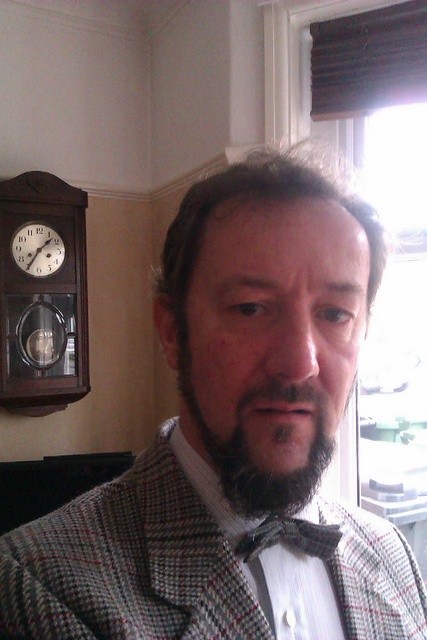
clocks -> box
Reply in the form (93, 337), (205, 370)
(0, 169), (91, 411)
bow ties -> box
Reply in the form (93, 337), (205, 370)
(234, 517), (343, 563)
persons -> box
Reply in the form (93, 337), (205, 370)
(0, 136), (426, 640)
